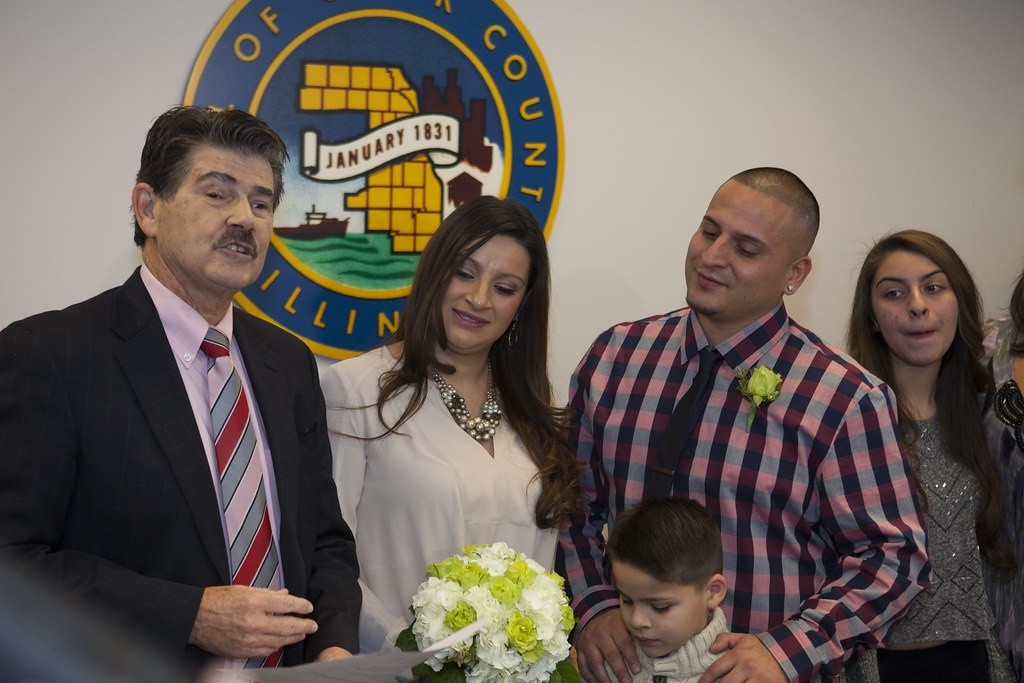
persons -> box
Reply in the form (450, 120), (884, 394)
(579, 494), (733, 683)
(0, 105), (364, 683)
(320, 194), (583, 683)
(846, 229), (1024, 683)
(554, 166), (935, 683)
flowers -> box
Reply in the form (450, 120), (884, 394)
(733, 364), (783, 435)
(393, 544), (583, 683)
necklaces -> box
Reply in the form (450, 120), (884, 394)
(429, 356), (503, 442)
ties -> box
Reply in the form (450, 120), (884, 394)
(641, 347), (724, 502)
(199, 327), (284, 669)
